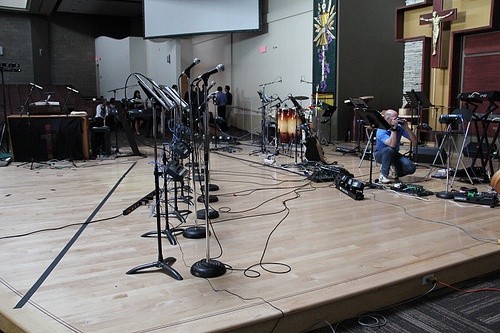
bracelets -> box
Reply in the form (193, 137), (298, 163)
(390, 128), (397, 131)
(405, 128), (410, 131)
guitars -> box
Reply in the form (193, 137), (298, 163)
(289, 97), (324, 161)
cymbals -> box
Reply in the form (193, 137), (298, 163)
(294, 96), (309, 100)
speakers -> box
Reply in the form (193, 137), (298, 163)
(463, 142), (497, 159)
(413, 147), (447, 164)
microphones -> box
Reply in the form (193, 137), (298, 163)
(30, 83), (43, 89)
(195, 64), (224, 81)
(67, 86), (79, 93)
(207, 81), (216, 91)
(198, 93), (215, 110)
(181, 58), (201, 76)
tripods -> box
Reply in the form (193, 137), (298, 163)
(344, 96), (374, 156)
(126, 75), (191, 280)
(354, 107), (395, 189)
(249, 80), (295, 159)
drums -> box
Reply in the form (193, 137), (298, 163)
(277, 107), (302, 144)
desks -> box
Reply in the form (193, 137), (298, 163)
(6, 114), (93, 162)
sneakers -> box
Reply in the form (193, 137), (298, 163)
(379, 173), (391, 184)
(389, 166), (398, 178)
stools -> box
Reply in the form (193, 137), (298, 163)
(425, 108), (474, 188)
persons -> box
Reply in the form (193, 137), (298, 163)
(172, 85), (177, 92)
(130, 90), (144, 135)
(216, 85), (232, 131)
(184, 84), (204, 117)
(95, 98), (120, 127)
(375, 109), (417, 184)
